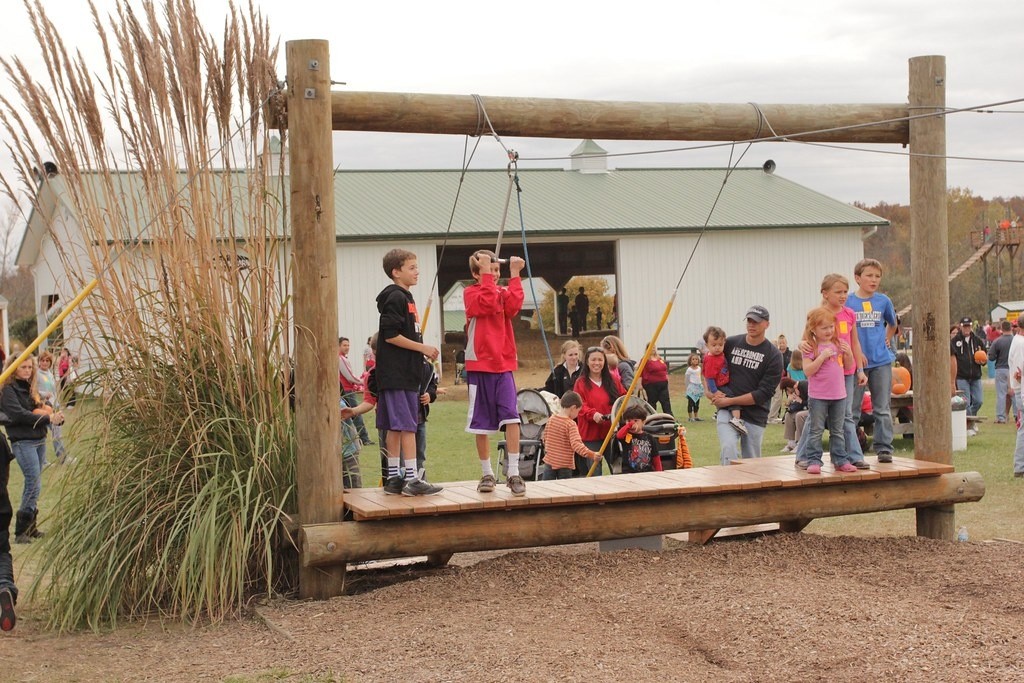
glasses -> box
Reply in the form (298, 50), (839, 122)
(586, 346), (604, 354)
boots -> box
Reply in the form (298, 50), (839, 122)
(27, 507), (45, 537)
(14, 510), (34, 544)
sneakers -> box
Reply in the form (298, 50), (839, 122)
(0, 587), (16, 631)
(383, 476), (405, 494)
(506, 474), (526, 496)
(477, 475), (496, 492)
(401, 468), (444, 497)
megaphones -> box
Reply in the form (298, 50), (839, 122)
(763, 160), (776, 174)
(33, 166), (43, 181)
(44, 162), (57, 178)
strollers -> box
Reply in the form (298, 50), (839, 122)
(494, 387), (560, 482)
(601, 394), (691, 473)
(453, 348), (468, 385)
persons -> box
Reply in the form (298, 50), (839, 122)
(0, 389), (18, 631)
(714, 304), (784, 465)
(0, 344), (6, 375)
(607, 294), (617, 329)
(846, 258), (898, 462)
(521, 339), (586, 478)
(35, 348), (79, 465)
(638, 342), (674, 416)
(0, 350), (64, 544)
(951, 314), (1024, 477)
(606, 352), (621, 381)
(802, 308), (858, 473)
(600, 335), (636, 473)
(281, 335), (437, 490)
(541, 389), (603, 481)
(700, 325), (748, 435)
(373, 248), (443, 497)
(463, 249), (526, 496)
(766, 309), (913, 452)
(684, 353), (705, 422)
(794, 273), (871, 469)
(616, 404), (662, 473)
(573, 346), (627, 476)
(557, 286), (590, 337)
(595, 307), (602, 330)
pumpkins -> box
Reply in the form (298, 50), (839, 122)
(974, 346), (986, 363)
(891, 361), (912, 395)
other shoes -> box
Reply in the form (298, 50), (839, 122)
(67, 405), (74, 409)
(687, 412), (800, 454)
(807, 464), (821, 474)
(43, 462), (54, 469)
(851, 459), (870, 469)
(65, 457), (77, 464)
(834, 462), (857, 472)
(967, 413), (1024, 477)
(729, 417), (748, 434)
(878, 451), (892, 462)
(794, 460), (808, 470)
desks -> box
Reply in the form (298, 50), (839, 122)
(863, 389), (963, 420)
(655, 347), (700, 375)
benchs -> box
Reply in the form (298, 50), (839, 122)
(655, 354), (701, 375)
(865, 411), (987, 436)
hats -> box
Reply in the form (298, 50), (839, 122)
(960, 316), (972, 326)
(743, 305), (769, 323)
